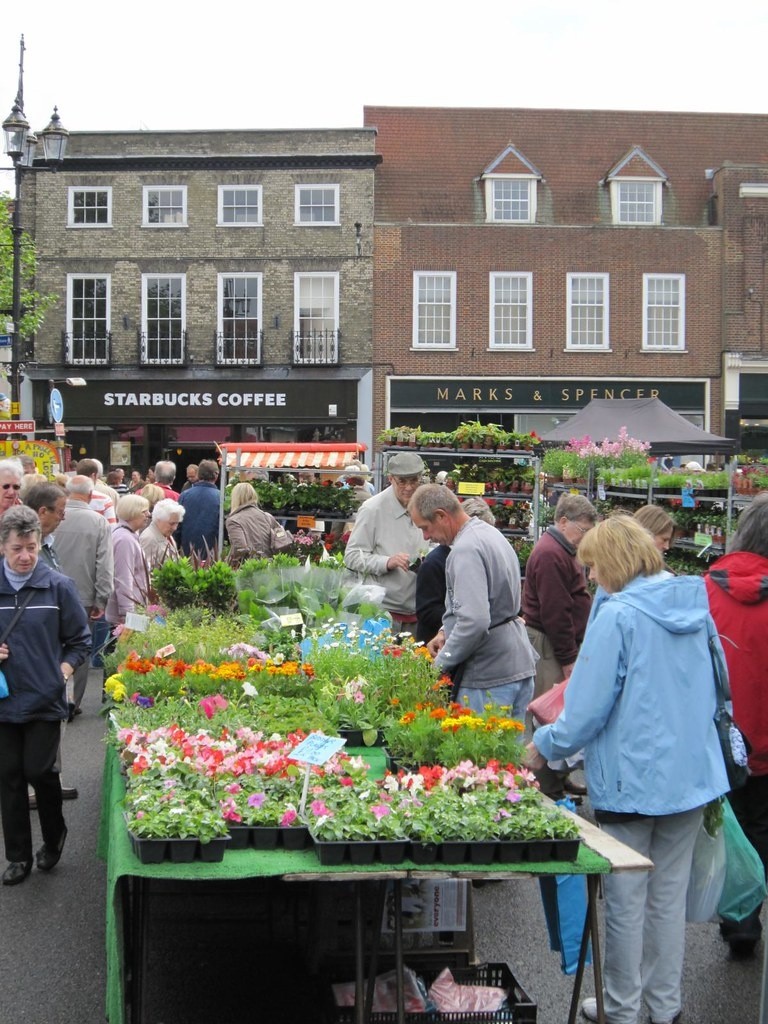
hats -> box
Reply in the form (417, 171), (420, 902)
(387, 452), (425, 476)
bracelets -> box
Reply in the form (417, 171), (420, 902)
(62, 673), (68, 680)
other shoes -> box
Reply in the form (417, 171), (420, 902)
(2, 857), (34, 886)
(582, 997), (599, 1021)
(68, 701), (78, 724)
(62, 787), (78, 801)
(28, 794), (37, 808)
(36, 825), (68, 870)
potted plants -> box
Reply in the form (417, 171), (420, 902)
(376, 420), (541, 451)
(663, 547), (725, 576)
(506, 537), (534, 566)
(446, 463), (536, 493)
(542, 448), (745, 545)
(223, 475), (366, 519)
(149, 534), (382, 616)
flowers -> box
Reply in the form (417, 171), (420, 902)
(564, 426), (652, 457)
(732, 454), (768, 495)
(290, 529), (352, 566)
(98, 604), (583, 867)
(530, 431), (543, 441)
(457, 496), (533, 518)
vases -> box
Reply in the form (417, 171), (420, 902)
(495, 518), (530, 530)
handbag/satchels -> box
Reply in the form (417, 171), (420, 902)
(685, 823), (727, 924)
(717, 795), (767, 922)
(713, 711), (753, 792)
(529, 678), (569, 725)
(270, 527), (295, 551)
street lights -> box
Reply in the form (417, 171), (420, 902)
(0, 92), (70, 441)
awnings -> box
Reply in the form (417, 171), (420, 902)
(213, 442), (368, 468)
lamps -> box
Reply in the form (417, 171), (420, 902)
(79, 444), (86, 454)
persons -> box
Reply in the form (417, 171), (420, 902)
(0, 505), (93, 886)
(523, 513), (750, 1024)
(345, 452), (431, 645)
(521, 493), (597, 805)
(588, 505), (678, 623)
(702, 490), (768, 943)
(225, 483), (284, 571)
(435, 470), (449, 485)
(415, 497), (495, 645)
(0, 455), (222, 810)
(299, 469), (315, 484)
(408, 483), (541, 745)
(335, 464), (374, 511)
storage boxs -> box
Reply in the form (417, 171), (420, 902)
(327, 964), (538, 1024)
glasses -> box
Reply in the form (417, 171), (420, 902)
(392, 476), (420, 488)
(46, 507), (66, 518)
(1, 483), (21, 490)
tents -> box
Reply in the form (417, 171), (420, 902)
(534, 397), (743, 556)
